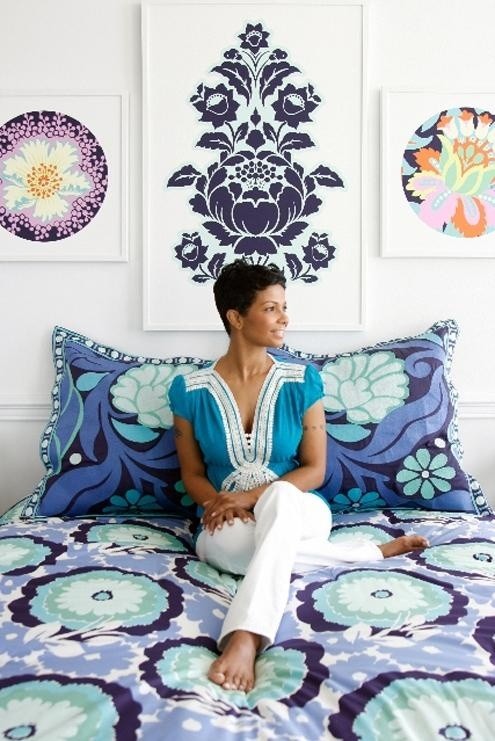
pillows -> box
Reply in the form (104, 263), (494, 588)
(17, 323), (212, 523)
(278, 319), (492, 517)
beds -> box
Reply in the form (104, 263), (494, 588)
(0, 480), (495, 741)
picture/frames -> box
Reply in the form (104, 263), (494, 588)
(0, 92), (130, 266)
(378, 84), (495, 261)
(140, 0), (369, 335)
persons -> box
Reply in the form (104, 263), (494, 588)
(167, 263), (431, 694)
